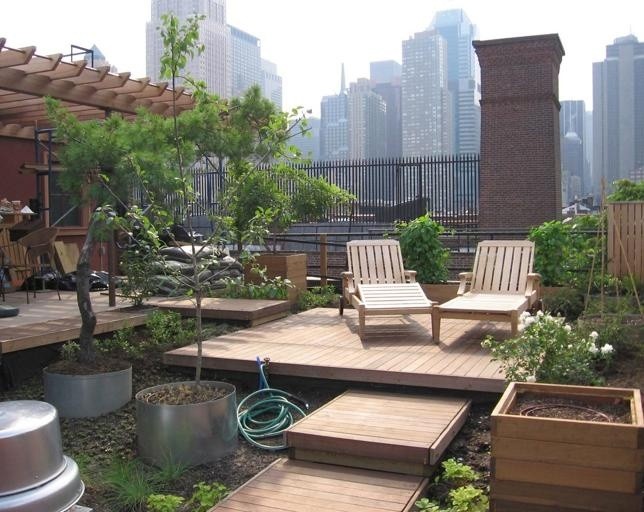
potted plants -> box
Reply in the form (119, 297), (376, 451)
(213, 164), (359, 304)
(488, 383), (644, 496)
(584, 272), (644, 313)
(381, 215), (578, 311)
(88, 12), (295, 470)
(577, 291), (644, 333)
(45, 88), (318, 420)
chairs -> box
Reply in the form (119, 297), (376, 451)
(339, 239), (439, 342)
(433, 239), (545, 346)
(0, 226), (61, 304)
